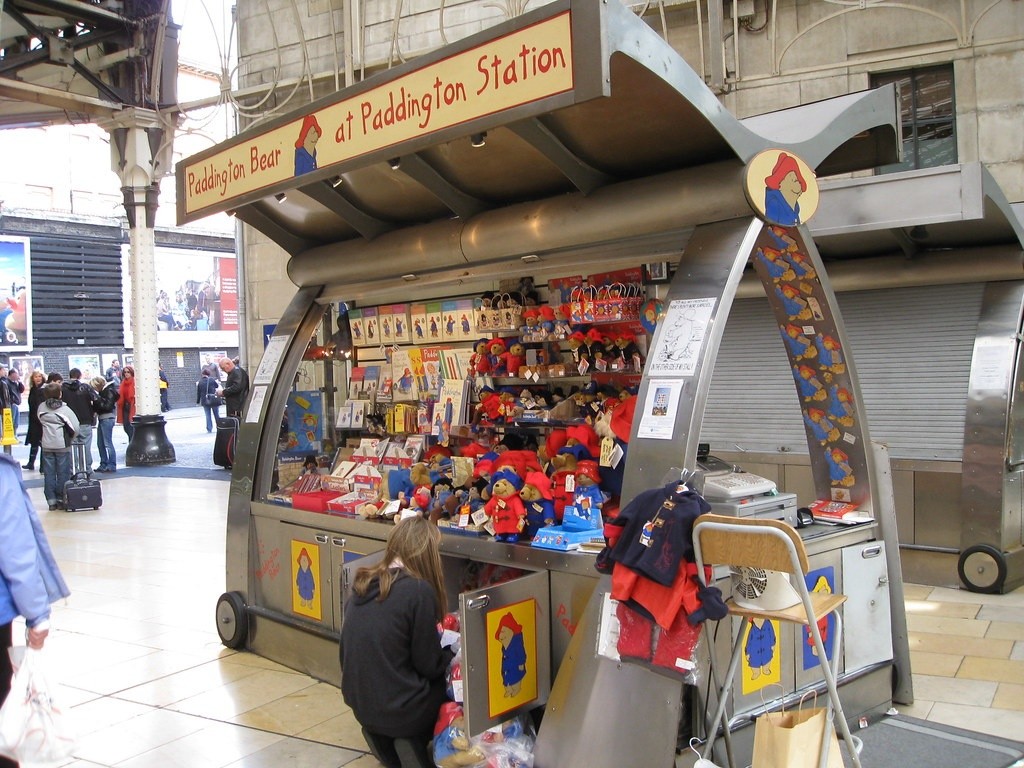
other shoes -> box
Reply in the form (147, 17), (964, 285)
(103, 466), (117, 472)
(23, 464), (35, 470)
(394, 737), (425, 768)
(48, 505), (57, 511)
(94, 466), (106, 472)
(58, 502), (67, 510)
(361, 725), (393, 766)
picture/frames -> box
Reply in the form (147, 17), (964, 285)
(641, 261), (671, 286)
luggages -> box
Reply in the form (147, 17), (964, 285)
(213, 408), (242, 469)
(63, 442), (102, 513)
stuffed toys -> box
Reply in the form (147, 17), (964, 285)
(357, 277), (645, 543)
(430, 558), (533, 768)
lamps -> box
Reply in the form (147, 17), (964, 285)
(327, 175), (343, 188)
(275, 193), (287, 204)
(387, 157), (401, 170)
(471, 130), (488, 147)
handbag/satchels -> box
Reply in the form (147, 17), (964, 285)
(751, 683), (846, 768)
(0, 617), (75, 768)
(205, 394), (222, 407)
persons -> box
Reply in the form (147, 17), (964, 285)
(156, 278), (220, 331)
(159, 362), (171, 412)
(216, 357), (249, 417)
(0, 451), (71, 768)
(202, 354), (220, 379)
(196, 369), (220, 432)
(12, 360), (41, 386)
(106, 359), (124, 392)
(0, 364), (135, 479)
(37, 383), (80, 510)
(338, 516), (461, 768)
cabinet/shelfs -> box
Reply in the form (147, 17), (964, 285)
(475, 316), (643, 430)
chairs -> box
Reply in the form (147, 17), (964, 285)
(692, 514), (863, 768)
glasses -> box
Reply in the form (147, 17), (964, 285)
(123, 371), (130, 374)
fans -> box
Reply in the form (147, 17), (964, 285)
(728, 562), (803, 611)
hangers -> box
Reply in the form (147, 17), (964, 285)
(689, 737), (721, 768)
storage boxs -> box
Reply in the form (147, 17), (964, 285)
(548, 364), (573, 377)
(519, 366), (530, 379)
(530, 366), (548, 378)
